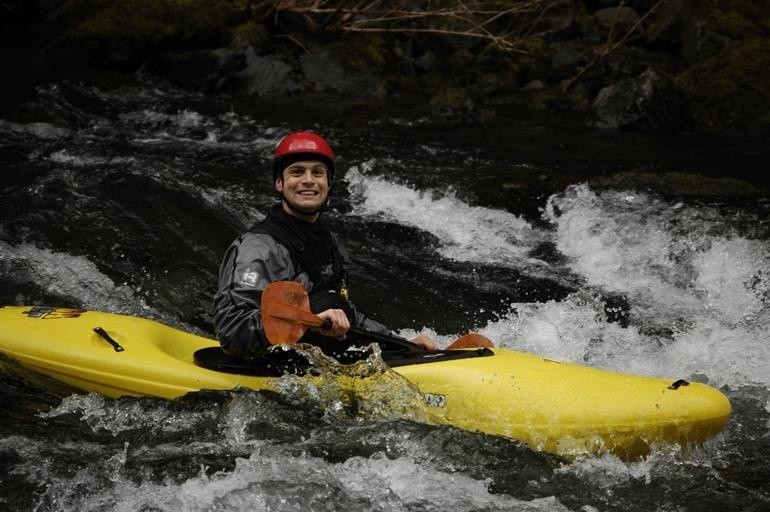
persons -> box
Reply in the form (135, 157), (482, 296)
(213, 132), (436, 356)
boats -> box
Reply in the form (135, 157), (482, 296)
(0, 305), (733, 463)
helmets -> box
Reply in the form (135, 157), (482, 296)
(272, 130), (336, 181)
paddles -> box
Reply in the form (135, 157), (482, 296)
(260, 280), (494, 351)
